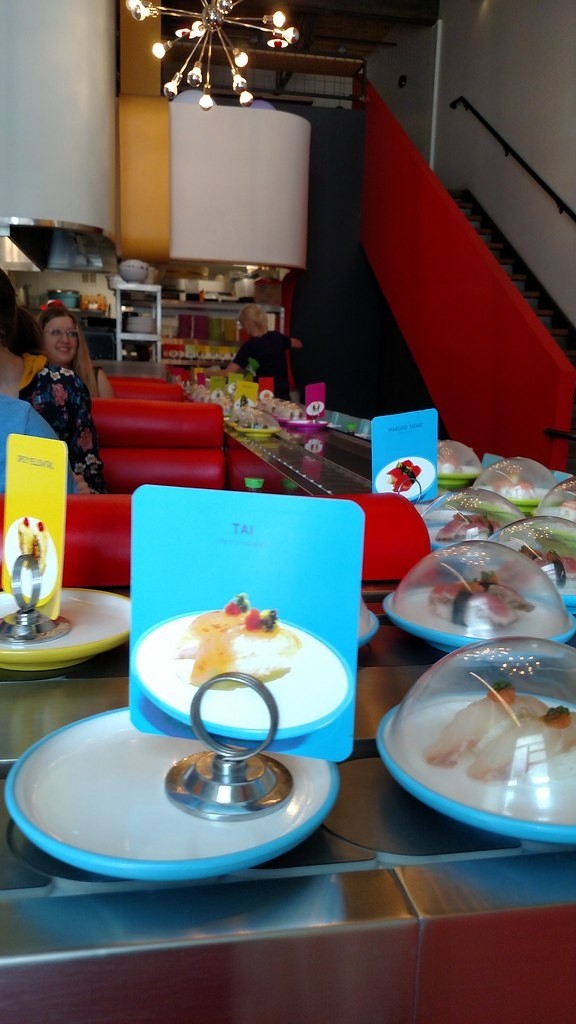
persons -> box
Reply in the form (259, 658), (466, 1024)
(201, 304), (303, 402)
(0, 268), (111, 495)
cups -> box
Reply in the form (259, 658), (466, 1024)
(224, 315), (238, 342)
(210, 317), (223, 342)
(195, 314), (209, 341)
(177, 313), (193, 339)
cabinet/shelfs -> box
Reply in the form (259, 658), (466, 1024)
(160, 300), (285, 373)
(115, 282), (161, 364)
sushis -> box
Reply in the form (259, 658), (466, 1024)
(175, 595), (250, 660)
(495, 483), (513, 497)
(429, 582), (518, 628)
(29, 522), (48, 575)
(394, 464), (421, 492)
(466, 571), (535, 612)
(18, 518), (30, 566)
(560, 500), (576, 520)
(518, 545), (543, 560)
(386, 460), (413, 484)
(183, 381), (303, 429)
(537, 550), (576, 588)
(468, 705), (576, 782)
(457, 513), (501, 539)
(512, 482), (533, 498)
(424, 681), (549, 767)
(436, 515), (472, 543)
(191, 608), (299, 690)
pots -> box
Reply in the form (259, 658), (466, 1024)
(118, 258), (152, 286)
(47, 288), (79, 309)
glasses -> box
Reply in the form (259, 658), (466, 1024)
(43, 328), (78, 337)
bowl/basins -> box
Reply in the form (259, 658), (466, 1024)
(127, 318), (156, 334)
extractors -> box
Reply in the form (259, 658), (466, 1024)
(8, 221), (120, 274)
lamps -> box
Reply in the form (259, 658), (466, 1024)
(125, 0), (300, 112)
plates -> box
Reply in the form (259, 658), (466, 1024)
(506, 498), (541, 517)
(130, 610), (354, 741)
(437, 466), (483, 490)
(224, 417), (282, 438)
(3, 707), (338, 882)
(358, 596), (576, 653)
(374, 702), (576, 843)
(418, 505), (576, 613)
(273, 415), (329, 431)
(0, 587), (130, 671)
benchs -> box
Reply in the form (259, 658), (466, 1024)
(0, 492), (431, 599)
(110, 382), (183, 401)
(107, 377), (167, 387)
(91, 399), (226, 493)
